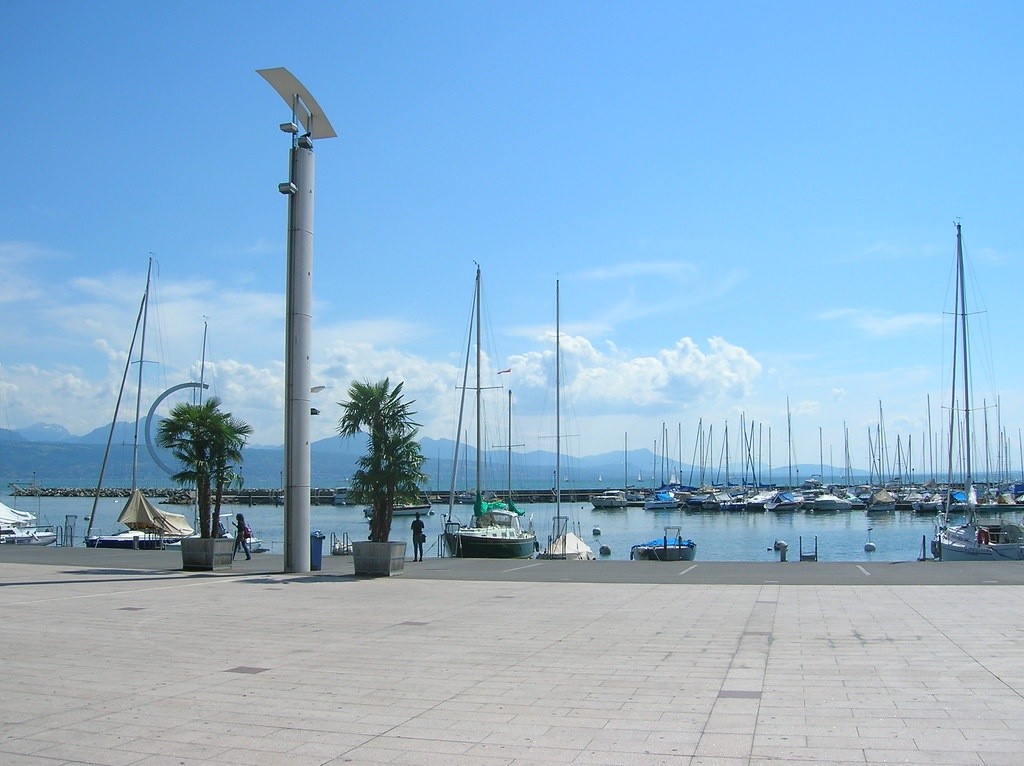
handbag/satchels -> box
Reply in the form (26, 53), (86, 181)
(422, 534), (426, 543)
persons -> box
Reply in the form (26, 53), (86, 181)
(410, 512), (425, 562)
(367, 509), (374, 540)
(232, 513), (251, 560)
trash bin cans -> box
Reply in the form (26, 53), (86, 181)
(310, 529), (326, 571)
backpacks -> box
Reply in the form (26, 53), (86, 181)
(244, 524), (252, 539)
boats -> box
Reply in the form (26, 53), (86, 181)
(632, 526), (696, 561)
(362, 496), (431, 517)
(0, 521), (57, 545)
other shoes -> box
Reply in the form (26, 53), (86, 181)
(419, 560), (422, 561)
(413, 560), (417, 562)
(246, 556), (251, 560)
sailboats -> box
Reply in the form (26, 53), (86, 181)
(83, 251), (193, 548)
(164, 315), (261, 553)
(766, 395), (942, 513)
(537, 279), (595, 560)
(933, 221), (1024, 559)
(942, 396), (1024, 511)
(454, 431), (479, 503)
(442, 261), (538, 558)
(589, 411), (777, 511)
